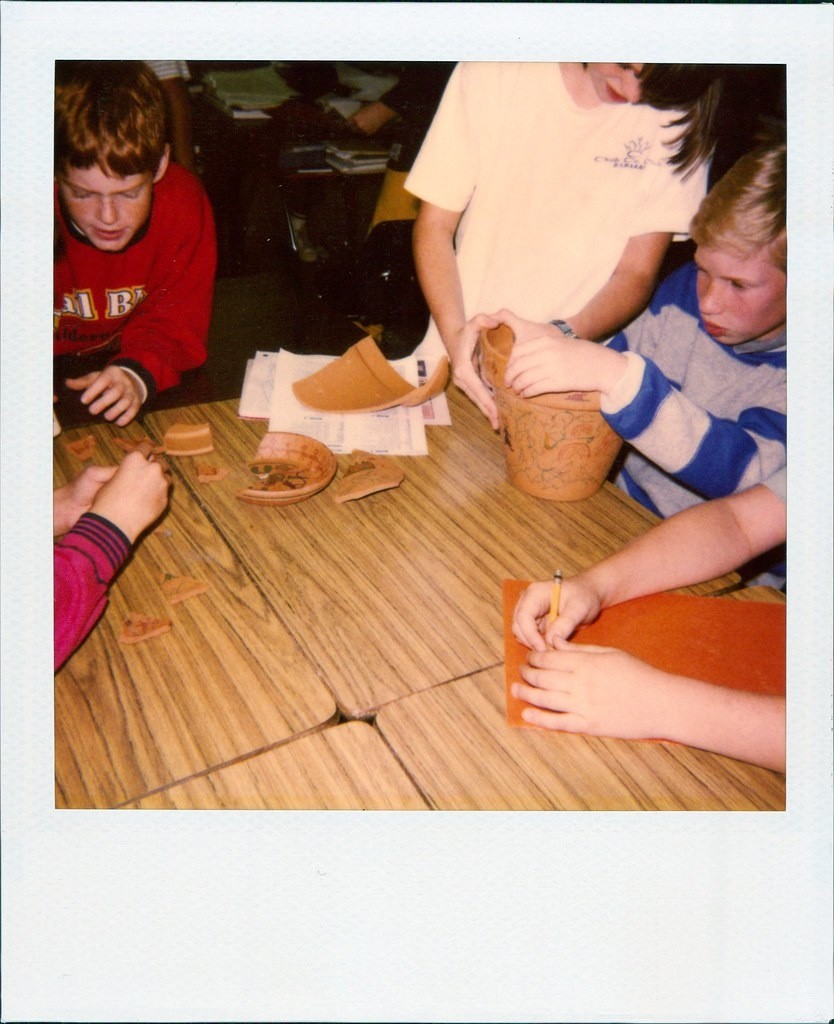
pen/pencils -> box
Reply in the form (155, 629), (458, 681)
(546, 569), (563, 651)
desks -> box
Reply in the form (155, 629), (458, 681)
(54, 384), (786, 811)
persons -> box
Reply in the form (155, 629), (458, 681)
(54, 60), (218, 437)
(287, 61), (457, 151)
(511, 466), (786, 776)
(53, 437), (173, 673)
(402, 62), (727, 430)
(505, 136), (786, 598)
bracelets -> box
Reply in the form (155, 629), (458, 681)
(548, 319), (581, 339)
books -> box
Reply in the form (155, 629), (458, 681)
(204, 94), (274, 119)
(324, 141), (389, 174)
